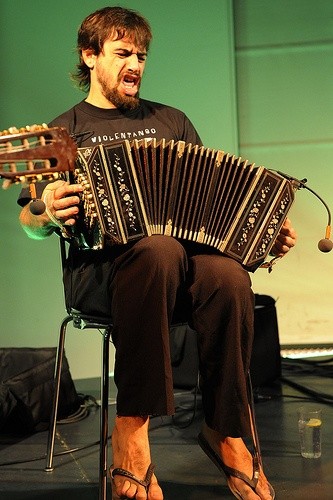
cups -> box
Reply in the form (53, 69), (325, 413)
(298, 408), (323, 458)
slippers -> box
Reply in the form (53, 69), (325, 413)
(196, 431), (276, 500)
(109, 463), (157, 500)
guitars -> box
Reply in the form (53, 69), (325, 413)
(0, 120), (95, 191)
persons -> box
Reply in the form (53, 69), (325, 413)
(17, 5), (296, 499)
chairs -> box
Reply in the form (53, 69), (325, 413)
(43, 234), (264, 500)
